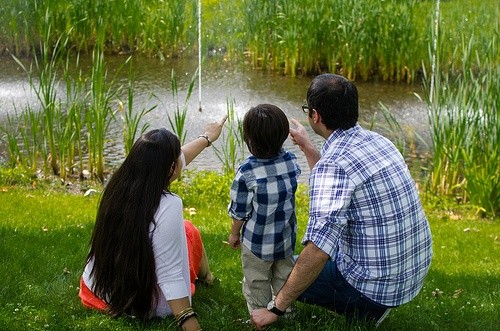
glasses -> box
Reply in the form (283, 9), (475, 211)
(302, 103), (313, 113)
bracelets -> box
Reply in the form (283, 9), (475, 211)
(199, 135), (212, 147)
(176, 308), (195, 326)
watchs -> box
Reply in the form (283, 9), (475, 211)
(267, 300), (286, 317)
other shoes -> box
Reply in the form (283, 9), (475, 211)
(198, 272), (214, 288)
(345, 296), (391, 329)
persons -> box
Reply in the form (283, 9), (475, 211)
(78, 114), (229, 331)
(229, 105), (302, 316)
(250, 74), (432, 331)
